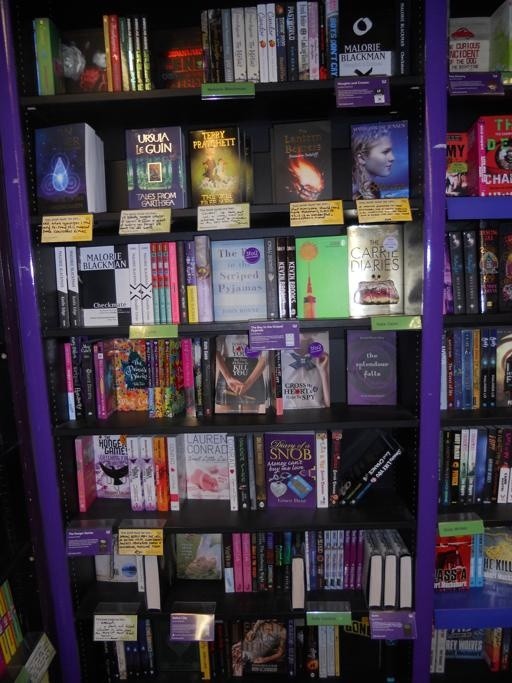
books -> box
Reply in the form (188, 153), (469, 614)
(0, 0), (511, 682)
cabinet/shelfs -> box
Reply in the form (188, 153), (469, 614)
(0, 0), (512, 683)
